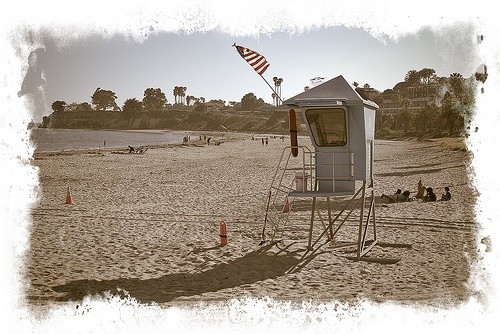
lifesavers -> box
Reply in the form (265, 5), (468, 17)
(289, 109), (298, 157)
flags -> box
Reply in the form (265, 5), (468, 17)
(236, 45), (270, 75)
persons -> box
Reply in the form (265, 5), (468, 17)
(423, 187), (436, 202)
(441, 187), (452, 200)
(183, 137), (188, 143)
(128, 145), (134, 154)
(266, 138), (269, 146)
(261, 138), (265, 146)
(282, 136), (284, 142)
(252, 135), (255, 141)
(380, 189), (412, 203)
(207, 137), (212, 146)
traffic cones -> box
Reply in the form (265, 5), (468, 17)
(64, 186), (74, 204)
